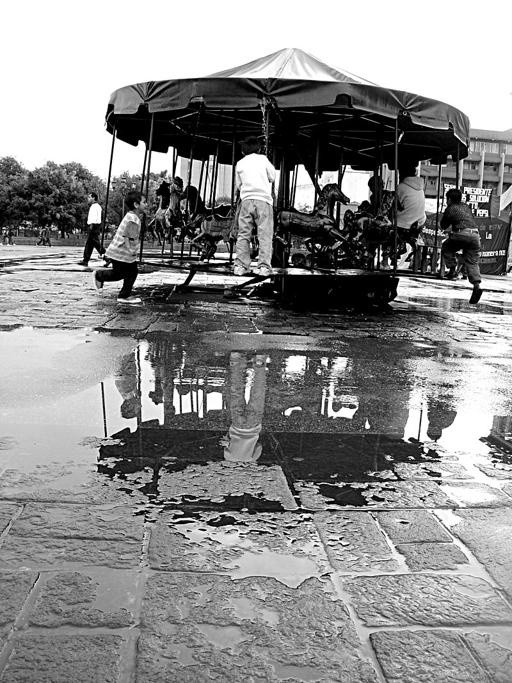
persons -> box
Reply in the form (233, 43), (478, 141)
(220, 352), (266, 462)
(233, 136), (277, 277)
(393, 161), (427, 255)
(92, 191), (148, 304)
(114, 353), (141, 419)
(76, 191), (112, 268)
(0, 218), (82, 246)
(427, 401), (457, 440)
(440, 189), (483, 304)
(355, 177), (393, 222)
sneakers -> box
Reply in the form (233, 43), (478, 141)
(469, 286), (482, 304)
(94, 271), (104, 290)
(117, 296), (141, 303)
(234, 266), (251, 275)
(259, 267), (272, 276)
(444, 270), (457, 277)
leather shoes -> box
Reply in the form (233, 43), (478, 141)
(77, 260), (88, 267)
(104, 260), (111, 267)
